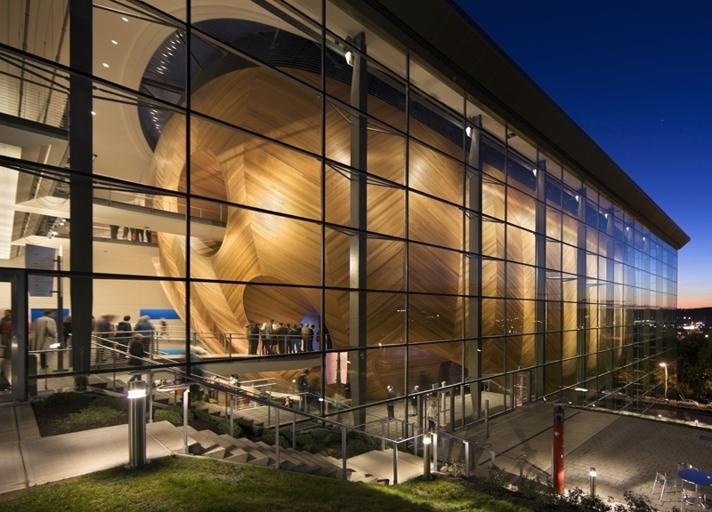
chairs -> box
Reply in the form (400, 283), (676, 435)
(652, 462), (712, 512)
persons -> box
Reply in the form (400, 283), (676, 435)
(127, 334), (151, 382)
(213, 374), (241, 388)
(344, 381), (351, 398)
(427, 401), (439, 433)
(109, 225), (153, 244)
(62, 314), (167, 363)
(31, 310), (58, 369)
(0, 310), (12, 383)
(297, 369), (310, 400)
(281, 396), (293, 408)
(247, 319), (327, 355)
(408, 385), (420, 415)
(386, 386), (397, 421)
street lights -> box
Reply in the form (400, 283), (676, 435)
(659, 363), (669, 399)
